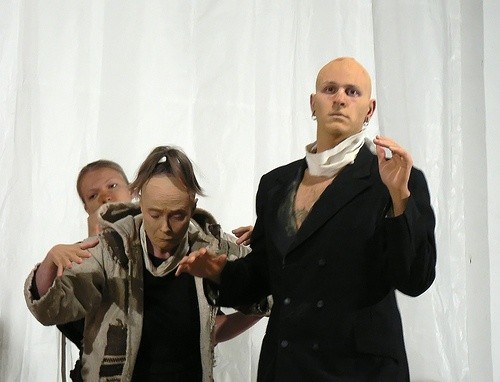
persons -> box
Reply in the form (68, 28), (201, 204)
(24, 58), (437, 382)
